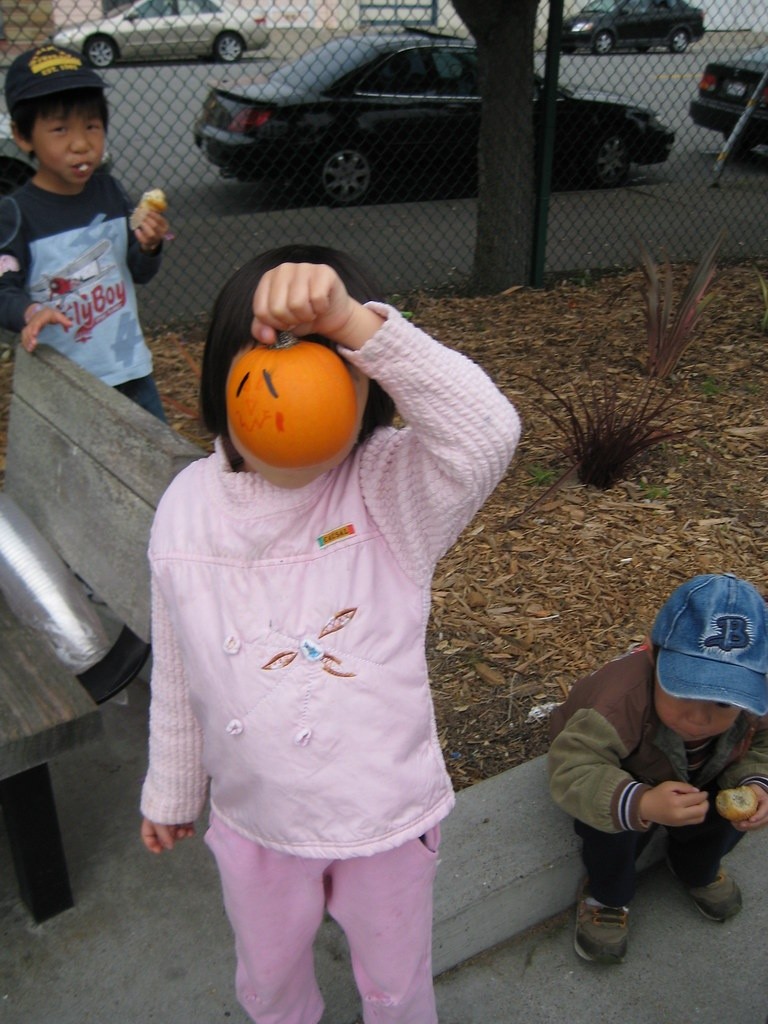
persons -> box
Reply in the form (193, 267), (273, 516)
(543, 571), (768, 966)
(135, 239), (523, 1024)
(0, 42), (163, 428)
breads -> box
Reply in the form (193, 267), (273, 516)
(716, 785), (758, 822)
(129, 189), (167, 231)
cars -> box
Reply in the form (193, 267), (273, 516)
(688, 43), (768, 152)
(191, 29), (678, 209)
(561, 0), (704, 55)
(1, 112), (114, 195)
(51, 0), (272, 70)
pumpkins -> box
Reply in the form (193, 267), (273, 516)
(225, 332), (356, 467)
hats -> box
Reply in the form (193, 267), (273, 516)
(652, 572), (768, 716)
(5, 43), (110, 111)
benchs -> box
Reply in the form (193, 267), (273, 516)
(0, 333), (207, 928)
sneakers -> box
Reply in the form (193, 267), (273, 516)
(574, 877), (629, 964)
(666, 857), (742, 923)
